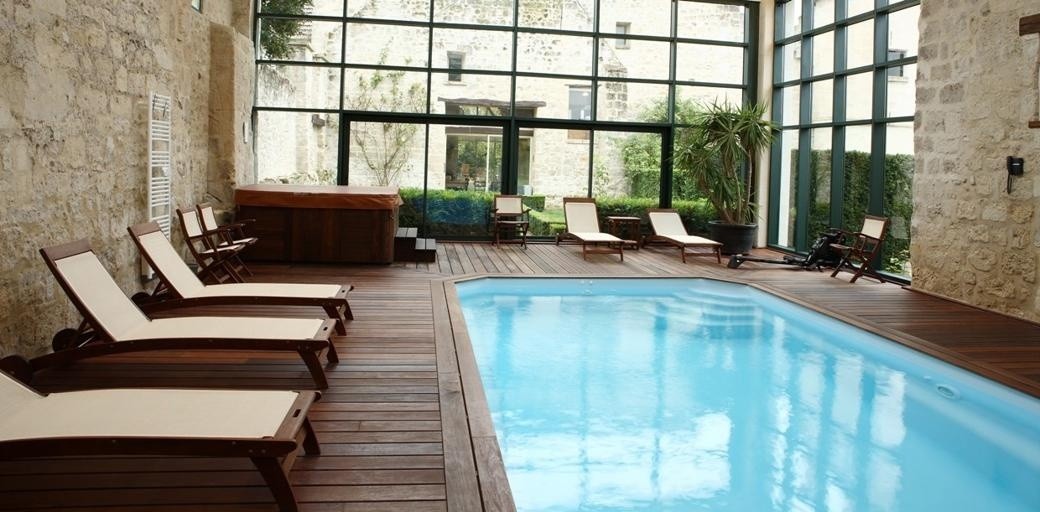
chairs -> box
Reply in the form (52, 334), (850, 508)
(1, 368), (327, 511)
(197, 202), (264, 280)
(128, 223), (356, 335)
(486, 193), (532, 250)
(642, 207), (723, 266)
(827, 214), (890, 284)
(556, 197), (626, 261)
(176, 208), (254, 284)
(39, 237), (340, 388)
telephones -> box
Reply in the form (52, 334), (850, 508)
(1006, 156), (1023, 176)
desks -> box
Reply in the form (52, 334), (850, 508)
(606, 215), (641, 250)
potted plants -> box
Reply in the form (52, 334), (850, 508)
(675, 88), (784, 257)
